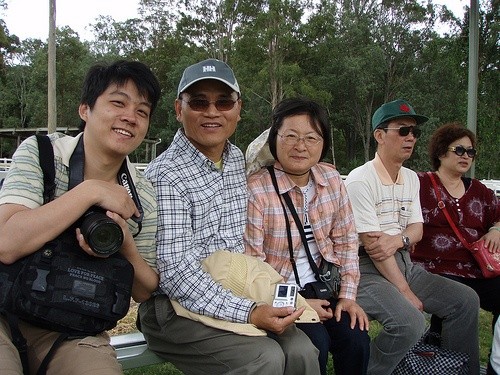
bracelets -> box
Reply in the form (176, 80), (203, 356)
(489, 227), (500, 231)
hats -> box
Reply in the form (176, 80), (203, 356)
(372, 99), (429, 131)
(176, 58), (241, 102)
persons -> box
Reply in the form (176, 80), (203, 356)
(143, 58), (320, 375)
(0, 60), (160, 375)
(343, 100), (480, 375)
(414, 123), (500, 375)
(244, 98), (370, 375)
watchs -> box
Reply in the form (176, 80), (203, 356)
(402, 235), (410, 245)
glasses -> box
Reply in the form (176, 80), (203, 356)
(276, 128), (324, 147)
(447, 146), (477, 158)
(179, 95), (241, 112)
(376, 126), (423, 139)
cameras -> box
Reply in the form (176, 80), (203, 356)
(273, 284), (298, 308)
(54, 205), (124, 256)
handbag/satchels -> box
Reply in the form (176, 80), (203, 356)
(469, 238), (500, 278)
(304, 260), (341, 311)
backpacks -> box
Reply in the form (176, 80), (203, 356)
(0, 134), (135, 375)
